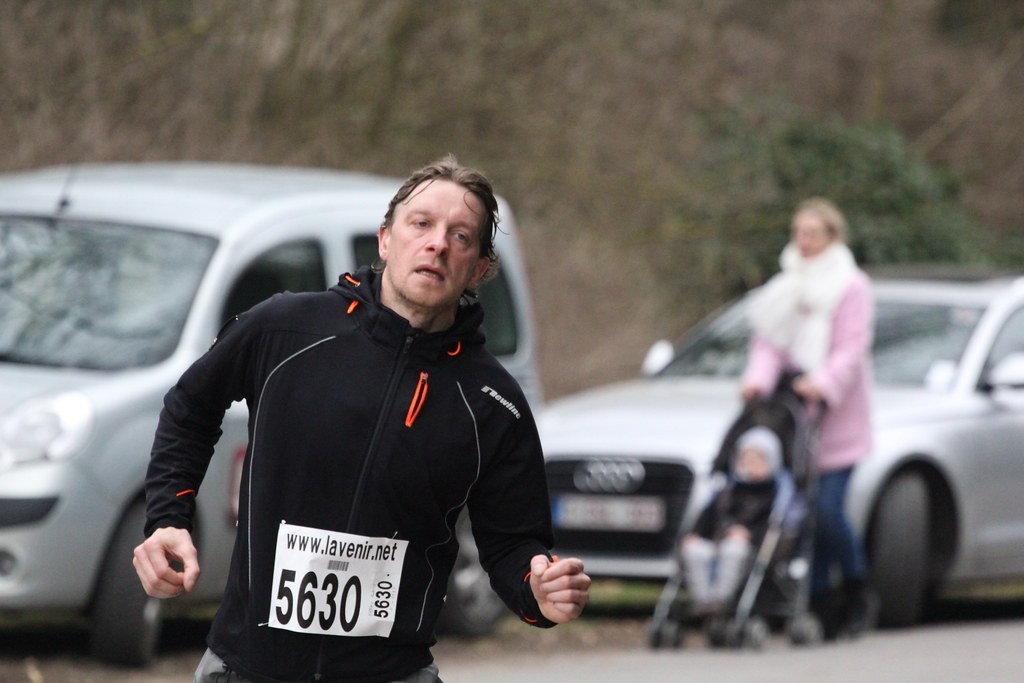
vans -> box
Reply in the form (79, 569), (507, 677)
(0, 147), (547, 674)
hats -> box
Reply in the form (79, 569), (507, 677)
(738, 425), (783, 473)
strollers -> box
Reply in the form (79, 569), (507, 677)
(643, 372), (827, 647)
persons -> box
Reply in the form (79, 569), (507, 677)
(681, 426), (785, 616)
(132, 155), (592, 683)
(741, 197), (874, 640)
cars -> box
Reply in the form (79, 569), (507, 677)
(534, 266), (1024, 629)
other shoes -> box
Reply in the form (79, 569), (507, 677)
(844, 575), (874, 633)
(810, 587), (838, 638)
(690, 595), (734, 616)
